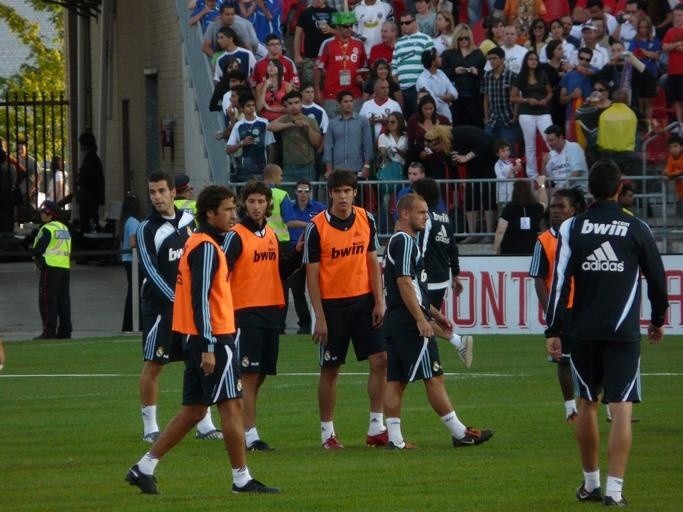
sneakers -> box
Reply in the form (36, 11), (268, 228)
(568, 412), (576, 423)
(453, 427), (492, 447)
(195, 429), (274, 450)
(143, 432), (159, 443)
(456, 336), (473, 368)
(232, 479), (280, 493)
(126, 465), (158, 493)
(576, 483), (627, 507)
(323, 430), (416, 449)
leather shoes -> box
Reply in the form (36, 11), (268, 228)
(34, 333), (70, 339)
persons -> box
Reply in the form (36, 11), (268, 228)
(121, 168), (491, 497)
(0, 336), (7, 369)
(185, 0), (682, 260)
(33, 201), (75, 338)
(0, 126), (108, 261)
(530, 161), (668, 505)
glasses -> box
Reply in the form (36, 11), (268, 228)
(297, 188), (309, 192)
(401, 20), (413, 25)
(579, 56), (589, 61)
(387, 120), (395, 123)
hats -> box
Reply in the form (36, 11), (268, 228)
(331, 13), (357, 26)
(581, 23), (596, 31)
(175, 175), (191, 190)
(40, 200), (60, 217)
